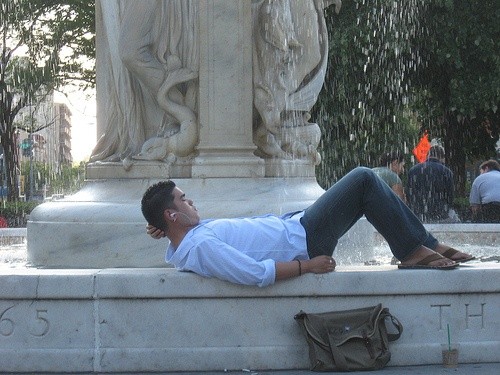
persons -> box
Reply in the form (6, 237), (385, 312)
(407, 145), (457, 222)
(141, 167), (476, 287)
(120, 0), (185, 137)
(373, 155), (406, 201)
(470, 160), (500, 223)
(251, 0), (329, 133)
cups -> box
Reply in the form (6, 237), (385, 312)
(441, 343), (461, 369)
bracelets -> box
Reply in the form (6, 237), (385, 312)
(296, 258), (301, 275)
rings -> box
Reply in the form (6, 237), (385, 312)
(329, 259), (332, 263)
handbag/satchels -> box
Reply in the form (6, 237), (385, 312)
(294, 302), (403, 372)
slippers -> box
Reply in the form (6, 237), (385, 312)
(398, 247), (475, 270)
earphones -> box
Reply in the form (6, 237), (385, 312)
(169, 213), (175, 218)
